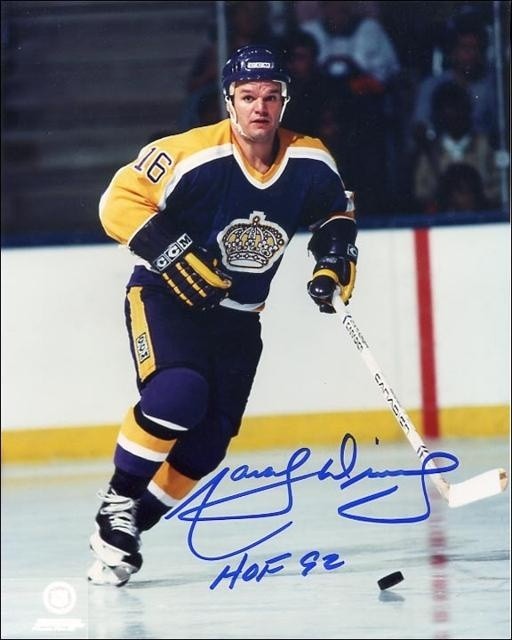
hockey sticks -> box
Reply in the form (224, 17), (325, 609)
(332, 285), (509, 510)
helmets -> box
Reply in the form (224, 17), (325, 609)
(221, 44), (292, 106)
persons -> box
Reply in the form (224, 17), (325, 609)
(94, 41), (359, 569)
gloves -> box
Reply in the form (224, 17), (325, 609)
(130, 213), (233, 313)
(307, 219), (359, 314)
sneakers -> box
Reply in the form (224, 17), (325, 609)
(93, 486), (142, 556)
(123, 533), (142, 568)
